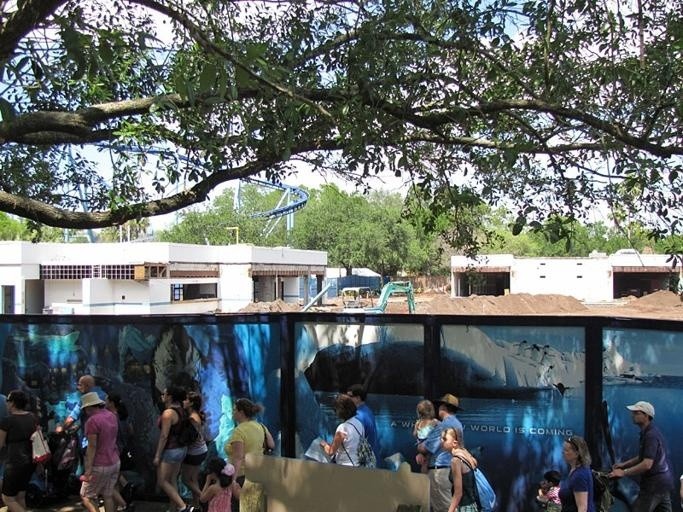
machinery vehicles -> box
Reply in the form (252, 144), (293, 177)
(340, 280), (415, 314)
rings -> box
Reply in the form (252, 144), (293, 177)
(610, 472), (613, 474)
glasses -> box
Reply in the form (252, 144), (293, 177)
(566, 436), (578, 450)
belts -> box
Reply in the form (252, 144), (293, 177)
(429, 466), (447, 469)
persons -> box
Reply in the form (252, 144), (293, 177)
(153, 385), (275, 511)
(556, 434), (595, 511)
(679, 474), (683, 510)
(534, 470), (563, 512)
(607, 399), (675, 511)
(413, 394), (478, 512)
(1, 375), (134, 512)
(320, 383), (376, 467)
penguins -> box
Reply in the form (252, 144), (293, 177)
(519, 340), (555, 378)
(552, 383), (570, 397)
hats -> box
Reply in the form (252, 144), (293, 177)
(347, 383), (368, 398)
(432, 393), (464, 411)
(626, 401), (654, 419)
(79, 391), (105, 410)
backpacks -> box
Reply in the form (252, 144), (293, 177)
(341, 421), (376, 468)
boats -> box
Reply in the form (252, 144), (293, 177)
(603, 373), (642, 386)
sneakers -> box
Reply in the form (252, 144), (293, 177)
(124, 481), (136, 500)
(176, 504), (195, 512)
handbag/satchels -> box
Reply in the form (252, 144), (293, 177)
(463, 450), (497, 512)
(31, 414), (53, 464)
(261, 423), (274, 456)
(173, 407), (199, 446)
(591, 469), (610, 512)
(120, 446), (138, 471)
(57, 430), (80, 473)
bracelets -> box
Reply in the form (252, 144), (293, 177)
(623, 468), (628, 475)
